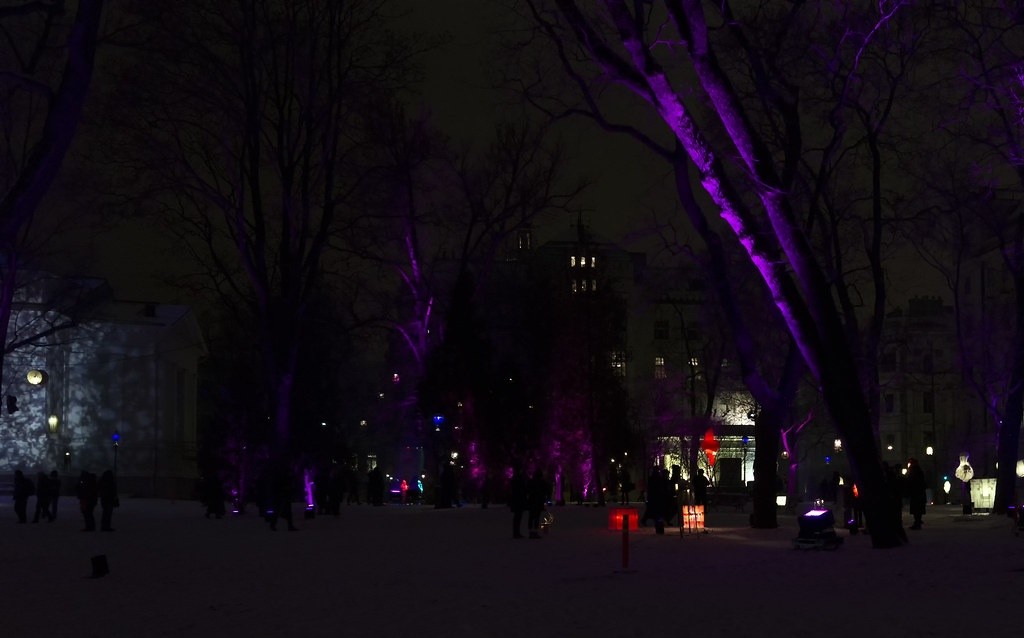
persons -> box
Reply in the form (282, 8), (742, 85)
(264, 466), (300, 531)
(13, 468), (61, 524)
(692, 468), (710, 514)
(642, 466), (675, 535)
(441, 462), (463, 508)
(843, 480), (864, 530)
(508, 463), (549, 540)
(344, 466), (387, 507)
(618, 467), (631, 505)
(199, 475), (226, 519)
(902, 458), (928, 530)
(78, 469), (120, 533)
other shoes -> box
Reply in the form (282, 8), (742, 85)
(288, 528), (299, 531)
(270, 525), (277, 531)
(528, 531), (542, 539)
(909, 525), (921, 530)
(102, 528), (115, 531)
(205, 513), (210, 519)
(81, 527), (95, 531)
(215, 516), (222, 519)
(918, 520), (924, 523)
(16, 510), (57, 524)
(513, 534), (523, 539)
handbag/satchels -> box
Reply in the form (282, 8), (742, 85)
(113, 498), (119, 507)
